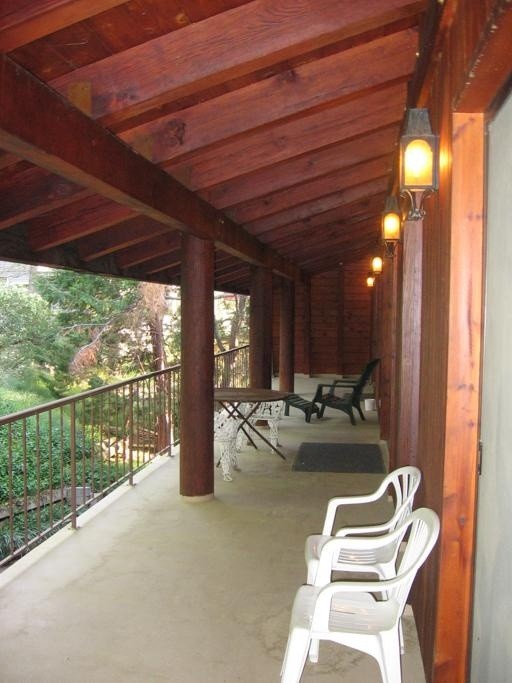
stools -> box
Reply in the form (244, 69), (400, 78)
(283, 392), (319, 421)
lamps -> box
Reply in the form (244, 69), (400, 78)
(378, 194), (401, 260)
(395, 104), (439, 221)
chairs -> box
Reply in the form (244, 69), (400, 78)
(279, 465), (441, 683)
(306, 356), (381, 425)
(212, 399), (286, 481)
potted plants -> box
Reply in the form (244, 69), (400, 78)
(361, 365), (377, 411)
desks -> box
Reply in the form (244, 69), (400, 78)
(214, 386), (290, 467)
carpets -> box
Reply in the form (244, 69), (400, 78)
(292, 441), (388, 474)
(342, 391), (374, 402)
